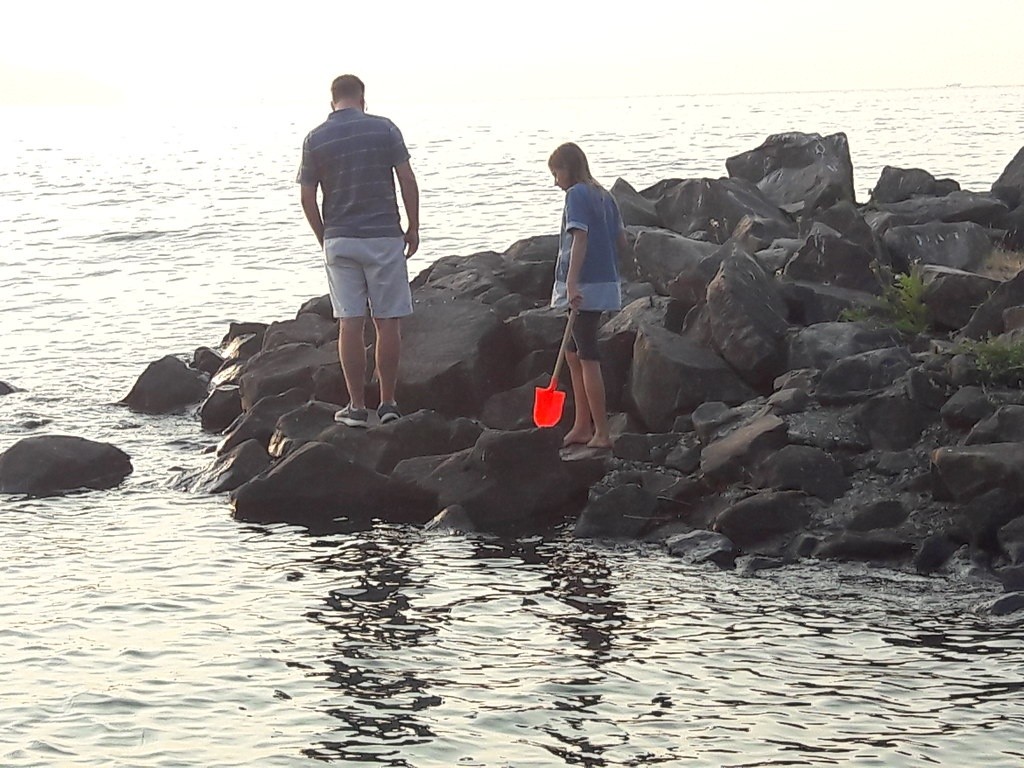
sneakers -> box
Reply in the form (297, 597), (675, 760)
(334, 401), (368, 427)
(377, 400), (401, 424)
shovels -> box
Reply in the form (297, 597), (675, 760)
(531, 293), (584, 429)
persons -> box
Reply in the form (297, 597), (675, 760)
(547, 142), (627, 449)
(296, 74), (420, 427)
